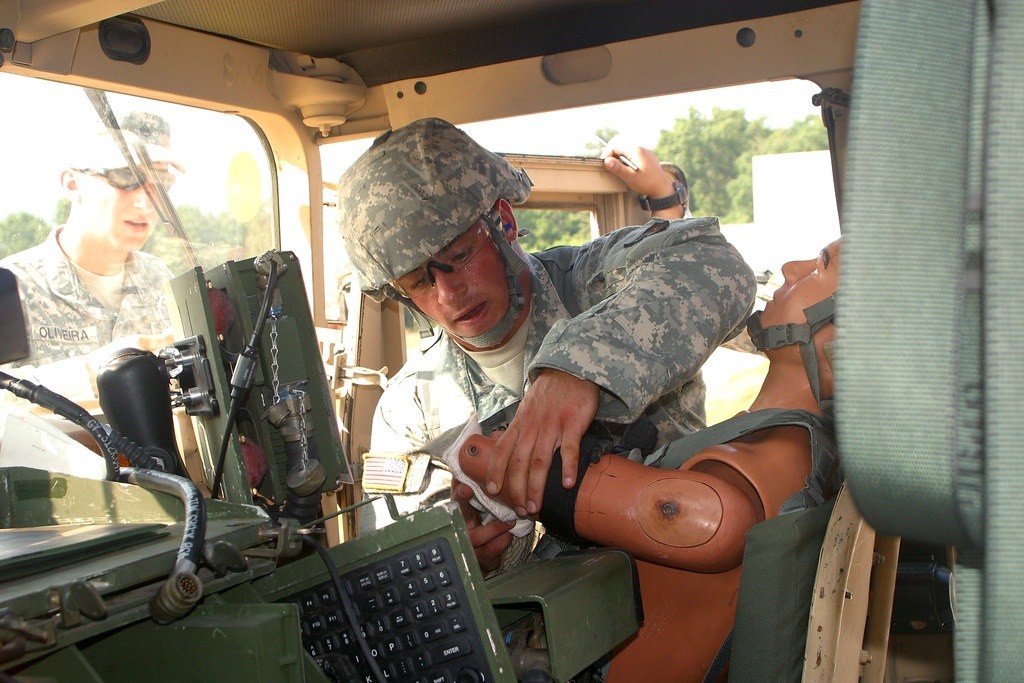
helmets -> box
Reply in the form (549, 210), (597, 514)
(338, 118), (534, 293)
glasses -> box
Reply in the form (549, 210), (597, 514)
(75, 161), (175, 193)
(394, 217), (493, 299)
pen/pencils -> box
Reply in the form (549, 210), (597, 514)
(594, 135), (640, 171)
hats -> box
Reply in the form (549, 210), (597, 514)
(65, 108), (187, 172)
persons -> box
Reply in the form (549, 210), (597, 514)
(337, 117), (757, 576)
(460, 230), (847, 683)
(599, 136), (779, 359)
(0, 109), (187, 414)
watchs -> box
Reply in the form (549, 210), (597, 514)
(638, 182), (688, 212)
